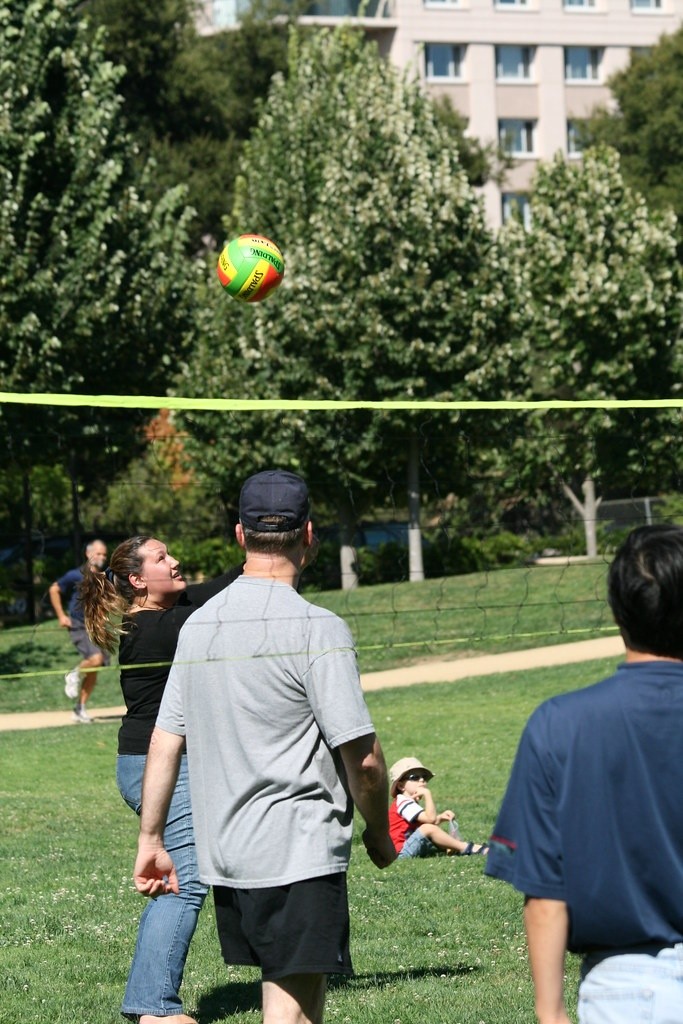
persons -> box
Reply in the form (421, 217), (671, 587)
(388, 757), (489, 858)
(133, 469), (399, 1024)
(73, 533), (322, 1024)
(486, 525), (683, 1024)
(49, 539), (114, 724)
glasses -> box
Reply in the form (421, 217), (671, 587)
(401, 774), (430, 782)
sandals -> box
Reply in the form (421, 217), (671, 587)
(456, 841), (490, 856)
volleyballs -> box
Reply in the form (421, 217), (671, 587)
(215, 234), (287, 305)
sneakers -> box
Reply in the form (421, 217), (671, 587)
(73, 704), (94, 724)
(64, 670), (86, 700)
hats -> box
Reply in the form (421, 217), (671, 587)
(387, 757), (435, 799)
(239, 470), (312, 532)
